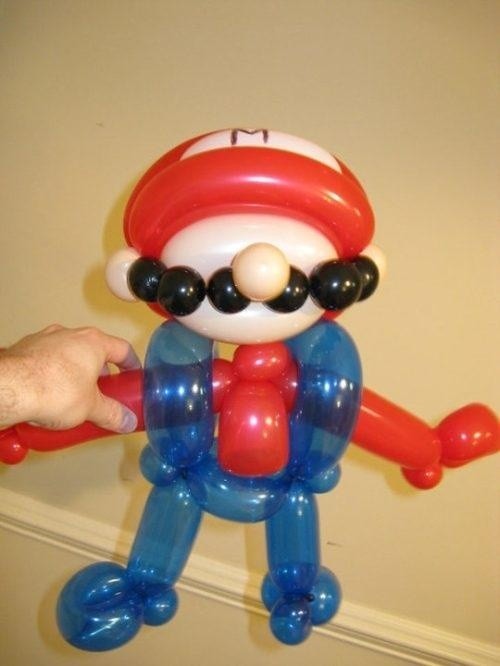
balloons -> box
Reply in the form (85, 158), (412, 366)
(0, 125), (499, 650)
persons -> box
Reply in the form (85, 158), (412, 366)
(0, 323), (143, 433)
